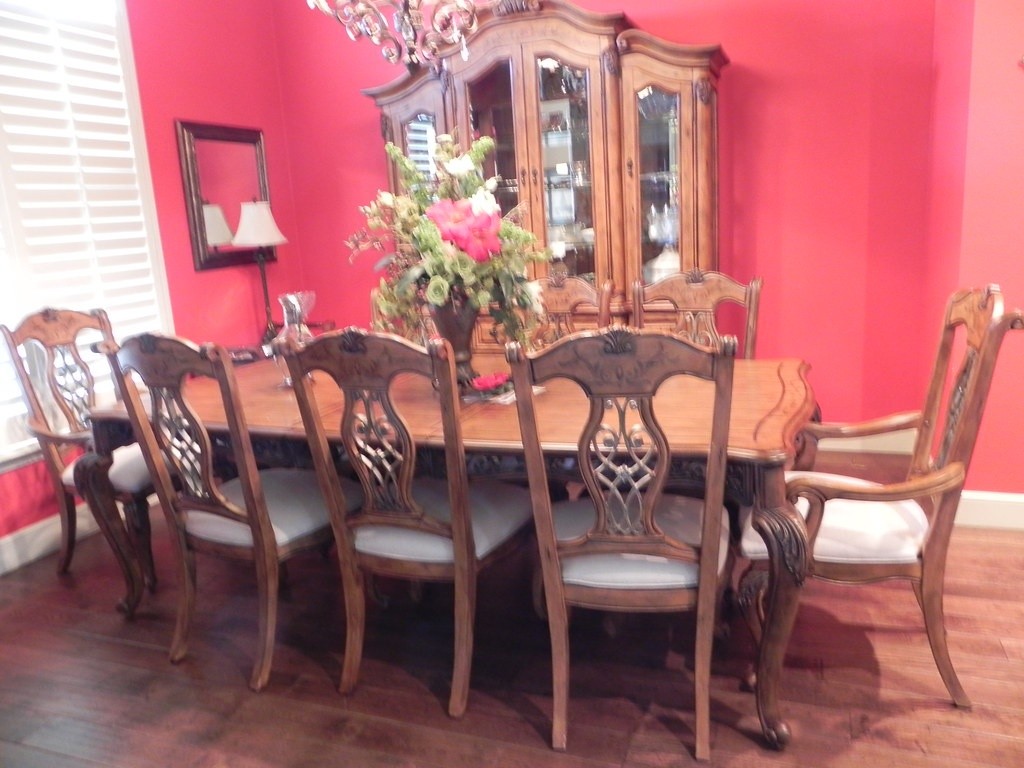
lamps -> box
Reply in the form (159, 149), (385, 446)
(201, 204), (232, 254)
(232, 196), (289, 348)
(306, 0), (538, 75)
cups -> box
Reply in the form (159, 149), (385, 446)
(570, 161), (587, 186)
(549, 223), (594, 242)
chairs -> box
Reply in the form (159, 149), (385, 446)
(740, 284), (1024, 707)
(510, 270), (614, 353)
(279, 325), (546, 721)
(90, 333), (367, 690)
(0, 307), (213, 592)
(632, 267), (762, 360)
(505, 325), (733, 766)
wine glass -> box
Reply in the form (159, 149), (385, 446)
(541, 112), (565, 135)
(571, 118), (588, 144)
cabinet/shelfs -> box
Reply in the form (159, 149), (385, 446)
(357, 1), (729, 347)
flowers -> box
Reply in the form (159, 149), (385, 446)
(342, 104), (551, 352)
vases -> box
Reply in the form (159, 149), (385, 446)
(422, 284), (483, 384)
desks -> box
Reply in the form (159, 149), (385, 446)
(90, 348), (819, 752)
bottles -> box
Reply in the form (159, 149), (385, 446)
(648, 172), (669, 202)
(649, 204), (672, 242)
(499, 176), (517, 187)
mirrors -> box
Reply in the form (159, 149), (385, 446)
(175, 119), (278, 273)
